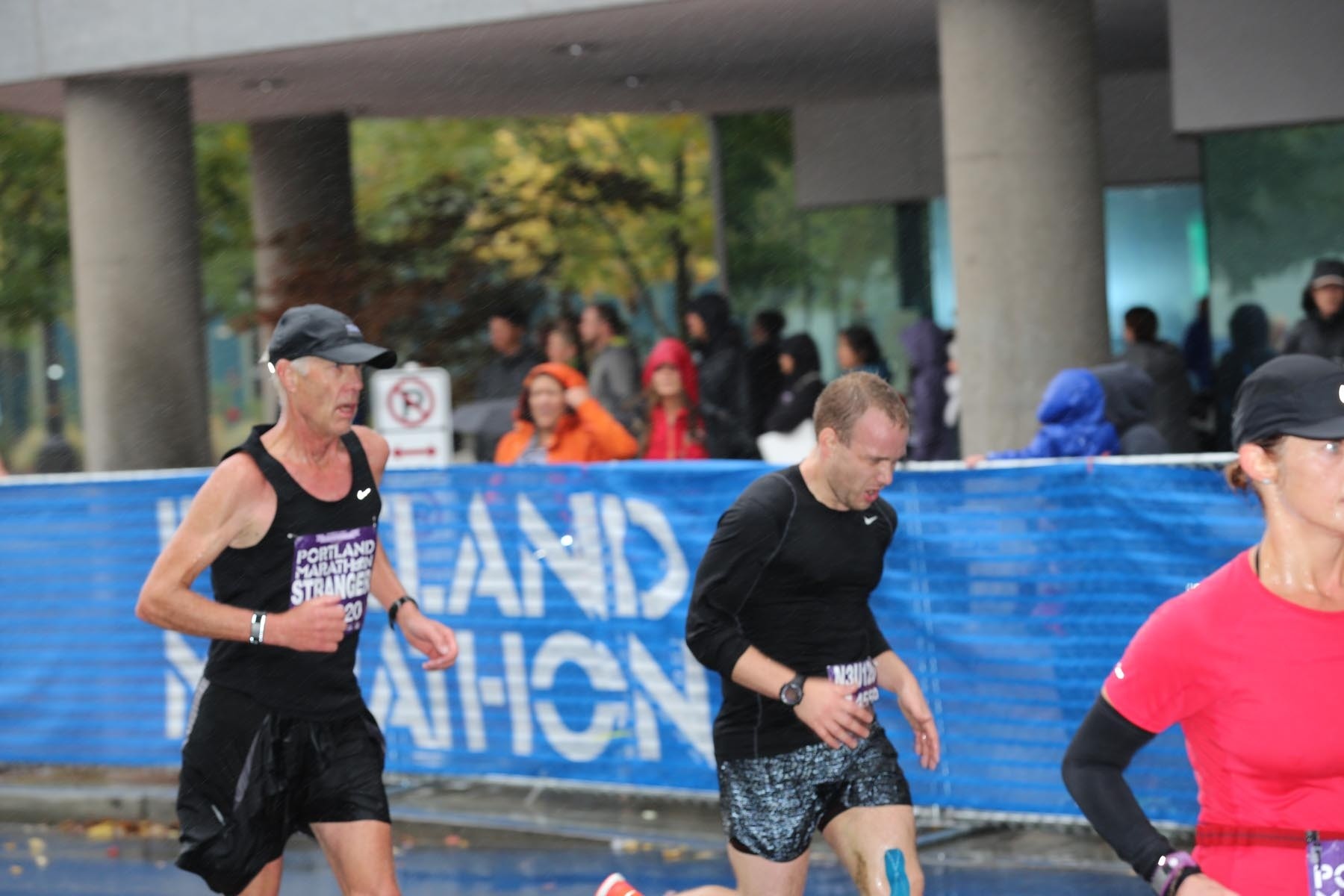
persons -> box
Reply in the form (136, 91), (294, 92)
(136, 304), (459, 896)
(480, 254), (1344, 466)
(595, 371), (941, 896)
(1061, 351), (1344, 896)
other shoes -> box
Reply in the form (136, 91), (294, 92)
(593, 871), (639, 896)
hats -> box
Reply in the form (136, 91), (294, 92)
(1313, 258), (1344, 290)
(1232, 355), (1343, 443)
(268, 303), (398, 370)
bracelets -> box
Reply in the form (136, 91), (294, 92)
(248, 611), (267, 645)
(1150, 849), (1200, 895)
(387, 596), (420, 630)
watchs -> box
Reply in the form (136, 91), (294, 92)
(779, 670), (808, 710)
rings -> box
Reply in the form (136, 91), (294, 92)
(921, 734), (926, 739)
